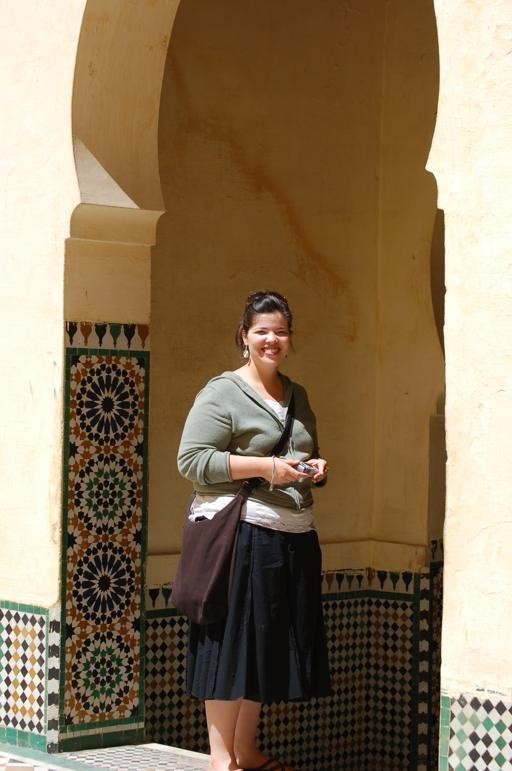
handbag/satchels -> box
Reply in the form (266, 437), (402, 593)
(171, 492), (244, 627)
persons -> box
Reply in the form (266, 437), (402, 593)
(175, 290), (333, 771)
